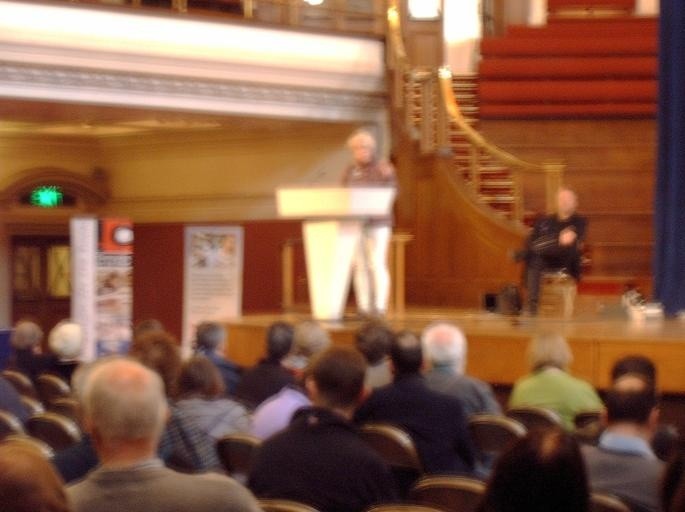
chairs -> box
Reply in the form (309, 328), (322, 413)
(36, 372), (71, 398)
(0, 411), (22, 437)
(456, 416), (528, 464)
(45, 396), (83, 420)
(410, 478), (490, 510)
(360, 424), (422, 478)
(536, 250), (585, 320)
(26, 413), (83, 452)
(16, 396), (42, 423)
(588, 493), (632, 512)
(3, 372), (34, 397)
(259, 497), (318, 512)
(211, 434), (263, 477)
(508, 407), (565, 438)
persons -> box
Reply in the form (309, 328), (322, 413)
(128, 331), (219, 470)
(512, 187), (589, 318)
(577, 370), (669, 505)
(343, 131), (396, 321)
(244, 344), (418, 511)
(177, 357), (250, 436)
(190, 321), (242, 397)
(511, 332), (603, 430)
(234, 320), (305, 408)
(471, 425), (591, 511)
(251, 358), (314, 442)
(420, 322), (506, 415)
(354, 331), (469, 471)
(62, 355), (261, 511)
